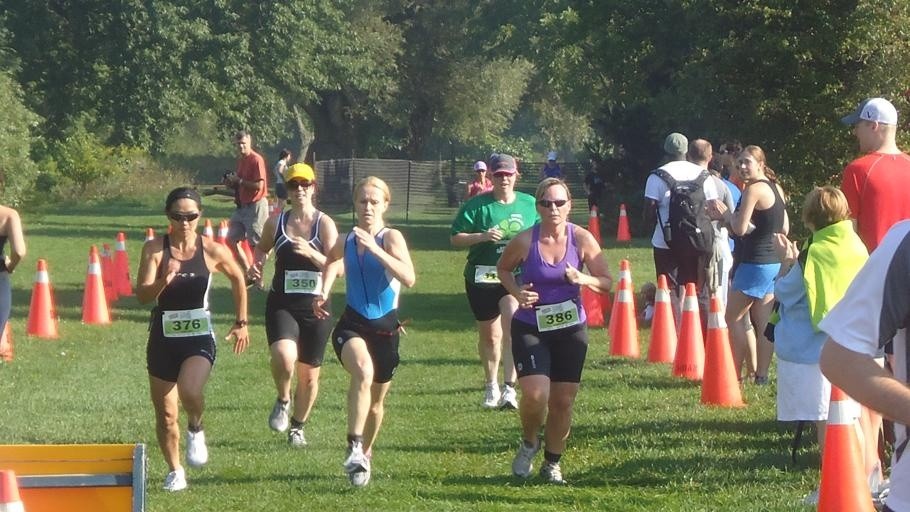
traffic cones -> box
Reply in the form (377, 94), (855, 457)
(79, 244), (111, 325)
(216, 218), (254, 264)
(100, 242), (119, 303)
(144, 228), (155, 241)
(579, 201), (741, 409)
(814, 384), (876, 511)
(202, 219), (214, 241)
(24, 258), (59, 338)
(268, 199), (274, 217)
(274, 201), (281, 214)
(0, 469), (25, 512)
(112, 233), (133, 297)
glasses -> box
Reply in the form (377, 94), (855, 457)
(169, 213), (198, 222)
(538, 200), (566, 208)
(287, 180), (311, 190)
(492, 172), (516, 178)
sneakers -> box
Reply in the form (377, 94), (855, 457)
(269, 398), (290, 432)
(287, 425), (307, 449)
(511, 438), (541, 476)
(539, 460), (562, 484)
(343, 441), (367, 474)
(185, 426), (208, 467)
(497, 384), (518, 410)
(482, 382), (500, 408)
(161, 465), (187, 492)
(349, 458), (370, 488)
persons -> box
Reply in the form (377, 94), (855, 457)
(583, 160), (609, 216)
(642, 133), (790, 384)
(636, 282), (656, 320)
(223, 130), (269, 292)
(272, 148), (291, 207)
(774, 185), (870, 507)
(537, 151), (566, 184)
(496, 177), (613, 484)
(450, 153), (542, 411)
(817, 219), (910, 511)
(463, 160), (493, 202)
(136, 187), (249, 491)
(0, 205), (27, 341)
(310, 176), (416, 486)
(839, 97), (910, 474)
(246, 162), (345, 447)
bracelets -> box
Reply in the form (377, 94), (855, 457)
(239, 178), (243, 184)
(235, 320), (249, 326)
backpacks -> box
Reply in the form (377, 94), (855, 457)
(653, 169), (715, 257)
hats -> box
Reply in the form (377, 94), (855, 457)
(488, 153), (516, 175)
(547, 152), (558, 160)
(284, 163), (316, 183)
(840, 98), (898, 125)
(474, 161), (487, 171)
